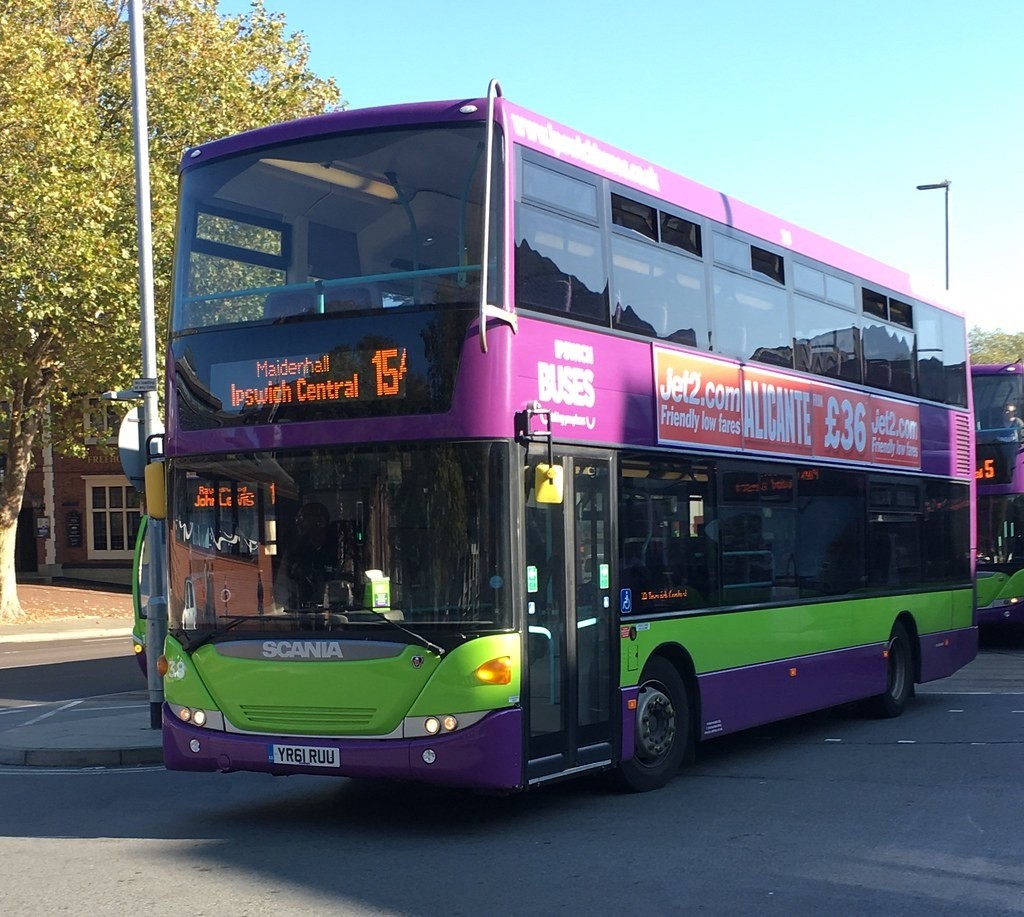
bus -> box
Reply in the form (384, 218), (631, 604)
(966, 356), (1023, 631)
(144, 77), (980, 795)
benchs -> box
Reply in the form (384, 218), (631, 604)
(709, 550), (775, 606)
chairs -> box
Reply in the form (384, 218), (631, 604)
(669, 586), (703, 610)
(263, 284), (315, 319)
(323, 283), (371, 312)
(555, 280), (914, 396)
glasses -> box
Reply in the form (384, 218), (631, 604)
(294, 514), (316, 525)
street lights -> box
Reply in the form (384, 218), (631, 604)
(915, 179), (952, 291)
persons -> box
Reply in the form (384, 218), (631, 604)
(993, 402), (1024, 429)
(274, 502), (478, 611)
